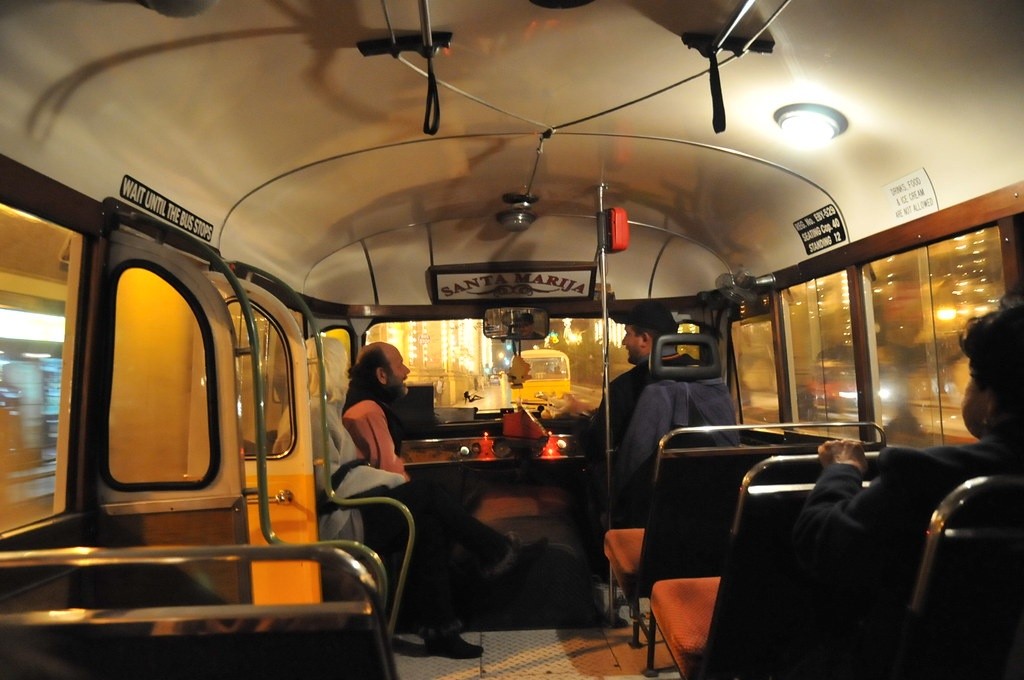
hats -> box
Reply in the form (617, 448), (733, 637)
(608, 301), (678, 332)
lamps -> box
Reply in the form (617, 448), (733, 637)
(772, 100), (851, 158)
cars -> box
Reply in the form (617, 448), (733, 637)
(807, 364), (891, 413)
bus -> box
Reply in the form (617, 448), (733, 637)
(511, 349), (570, 411)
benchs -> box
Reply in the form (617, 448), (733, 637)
(0, 539), (401, 680)
(888, 476), (1024, 680)
(646, 429), (965, 680)
(600, 416), (892, 647)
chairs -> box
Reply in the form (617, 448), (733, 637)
(610, 335), (736, 527)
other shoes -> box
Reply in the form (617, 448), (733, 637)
(422, 633), (484, 659)
(490, 537), (547, 599)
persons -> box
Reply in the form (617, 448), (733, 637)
(506, 312), (543, 339)
(342, 342), (512, 659)
(777, 306), (1024, 680)
(576, 299), (708, 527)
(434, 376), (445, 404)
